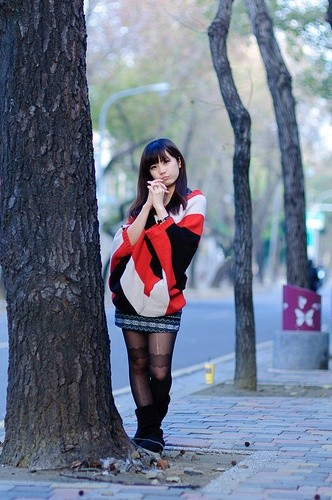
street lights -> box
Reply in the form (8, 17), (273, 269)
(95, 81), (173, 234)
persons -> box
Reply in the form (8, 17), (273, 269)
(108, 139), (208, 454)
(308, 259), (319, 292)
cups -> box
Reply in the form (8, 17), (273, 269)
(204, 363), (214, 385)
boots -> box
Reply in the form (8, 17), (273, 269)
(140, 396), (170, 453)
(131, 405), (152, 445)
